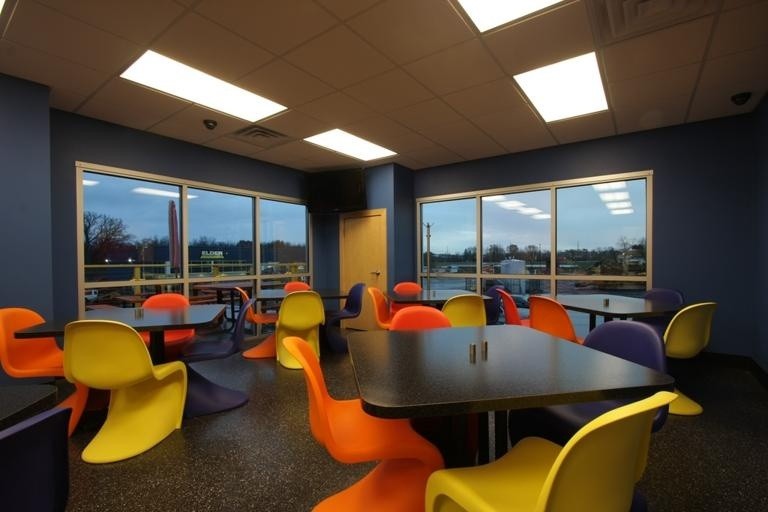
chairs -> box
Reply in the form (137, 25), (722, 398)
(137, 293), (195, 352)
(195, 281), (716, 414)
(1, 308), (89, 435)
(425, 389), (680, 510)
(187, 297), (256, 415)
(64, 321), (189, 464)
(279, 334), (442, 510)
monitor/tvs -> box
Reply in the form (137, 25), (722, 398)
(307, 176), (367, 213)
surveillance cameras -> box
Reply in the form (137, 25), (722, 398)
(730, 92), (752, 106)
(202, 119), (217, 130)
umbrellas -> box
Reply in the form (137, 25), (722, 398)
(168, 200), (181, 270)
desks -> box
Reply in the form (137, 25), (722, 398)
(15, 303), (225, 423)
(345, 324), (678, 464)
